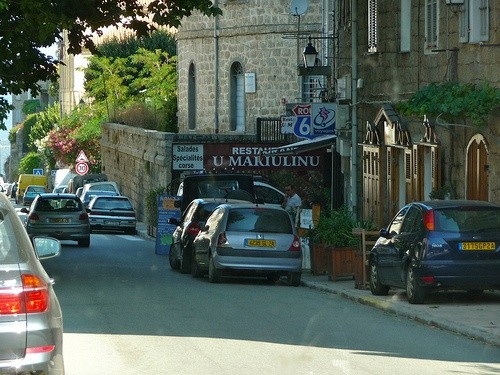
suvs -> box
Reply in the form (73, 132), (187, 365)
(0, 192), (67, 375)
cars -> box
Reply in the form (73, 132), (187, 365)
(20, 194), (90, 247)
(76, 181), (123, 208)
(0, 174), (46, 205)
(167, 174), (303, 287)
(369, 199), (500, 305)
(85, 195), (138, 235)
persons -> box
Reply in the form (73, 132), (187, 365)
(281, 184), (301, 231)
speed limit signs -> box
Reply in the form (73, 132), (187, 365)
(76, 163), (88, 175)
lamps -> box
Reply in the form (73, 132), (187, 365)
(302, 31), (339, 69)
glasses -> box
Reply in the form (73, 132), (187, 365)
(286, 189), (292, 191)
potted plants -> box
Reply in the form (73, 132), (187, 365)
(307, 208), (381, 289)
